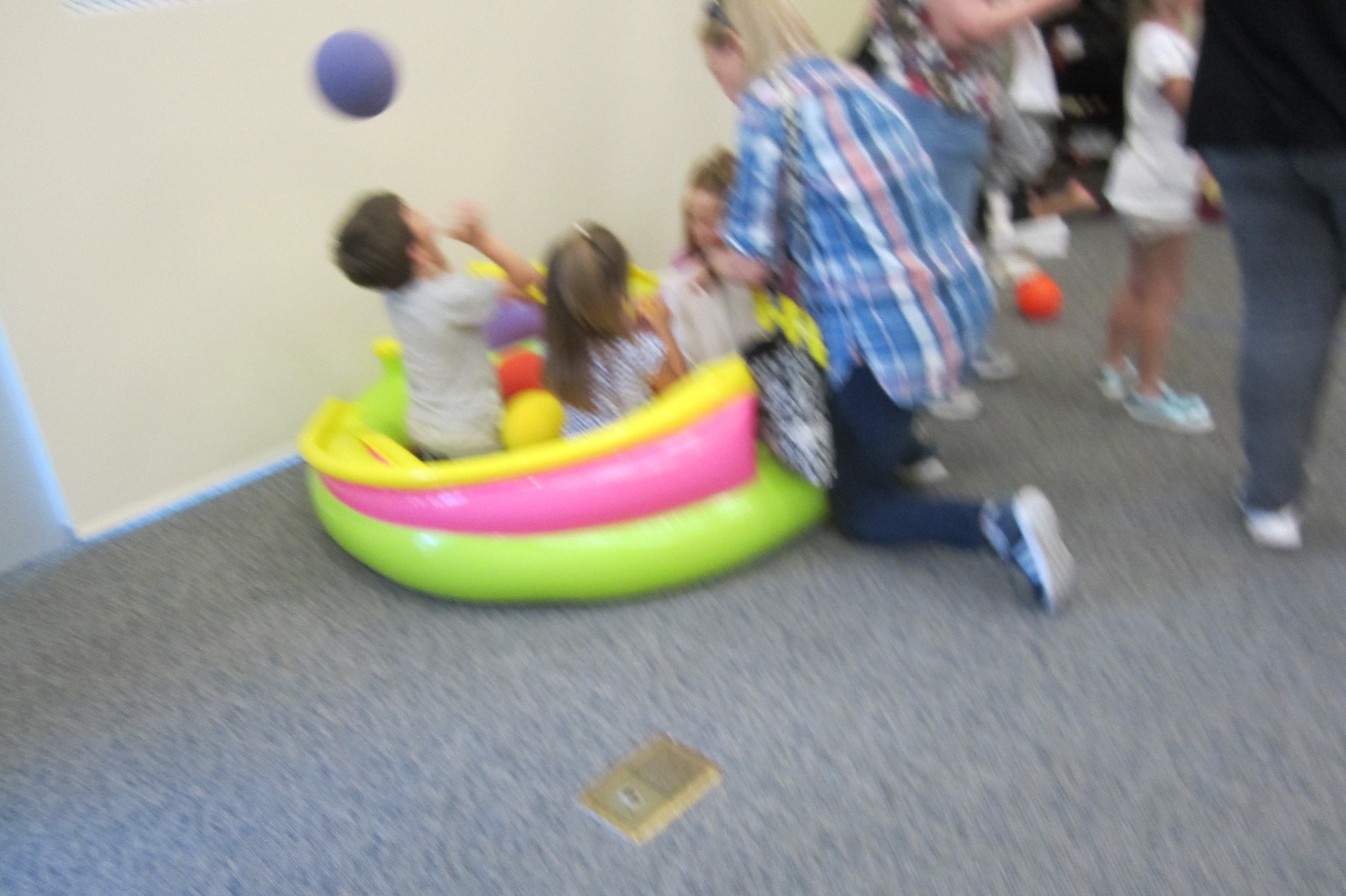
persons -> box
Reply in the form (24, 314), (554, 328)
(1184, 0), (1346, 553)
(669, 148), (745, 312)
(1101, 0), (1212, 434)
(927, 0), (1125, 252)
(690, 1), (1077, 612)
(334, 193), (545, 465)
(544, 221), (684, 439)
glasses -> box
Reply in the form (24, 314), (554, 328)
(709, 2), (736, 30)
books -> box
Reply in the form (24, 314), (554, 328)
(578, 736), (718, 846)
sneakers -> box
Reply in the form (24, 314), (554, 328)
(995, 484), (1075, 615)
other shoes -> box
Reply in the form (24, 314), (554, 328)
(901, 454), (949, 487)
(923, 384), (984, 421)
(1094, 354), (1144, 399)
(1236, 499), (1299, 549)
(1126, 380), (1215, 436)
(973, 344), (1015, 380)
